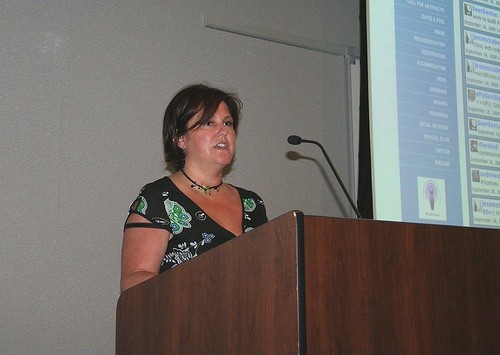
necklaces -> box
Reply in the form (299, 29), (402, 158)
(179, 167), (223, 195)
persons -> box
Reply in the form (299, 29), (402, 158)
(120, 83), (268, 294)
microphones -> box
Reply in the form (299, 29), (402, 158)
(287, 135), (361, 218)
